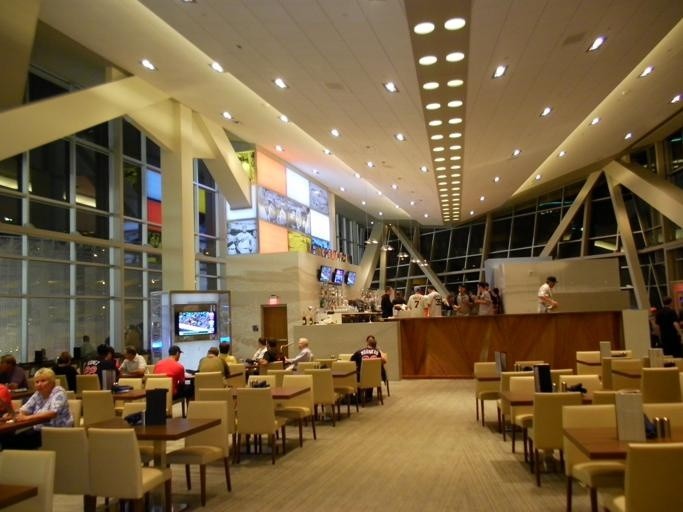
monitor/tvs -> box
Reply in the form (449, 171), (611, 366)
(174, 310), (218, 337)
(332, 268), (343, 286)
(344, 271), (356, 286)
(318, 265), (333, 283)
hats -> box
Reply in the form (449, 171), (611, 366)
(546, 276), (558, 283)
(168, 346), (183, 355)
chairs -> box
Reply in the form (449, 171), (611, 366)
(473, 350), (683, 512)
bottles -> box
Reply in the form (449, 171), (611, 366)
(551, 383), (556, 392)
(517, 363), (520, 371)
(512, 363), (516, 371)
(653, 417), (661, 438)
(561, 382), (567, 392)
(661, 416), (670, 438)
(300, 308), (319, 326)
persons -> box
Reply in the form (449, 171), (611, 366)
(197, 340), (238, 381)
(13, 367), (75, 449)
(250, 229), (258, 254)
(381, 282), (502, 318)
(350, 336), (387, 404)
(117, 343), (150, 376)
(234, 224), (254, 255)
(655, 297), (683, 358)
(226, 223), (239, 256)
(154, 344), (195, 401)
(0, 381), (15, 448)
(252, 337), (311, 372)
(537, 273), (559, 315)
(1, 335), (120, 391)
